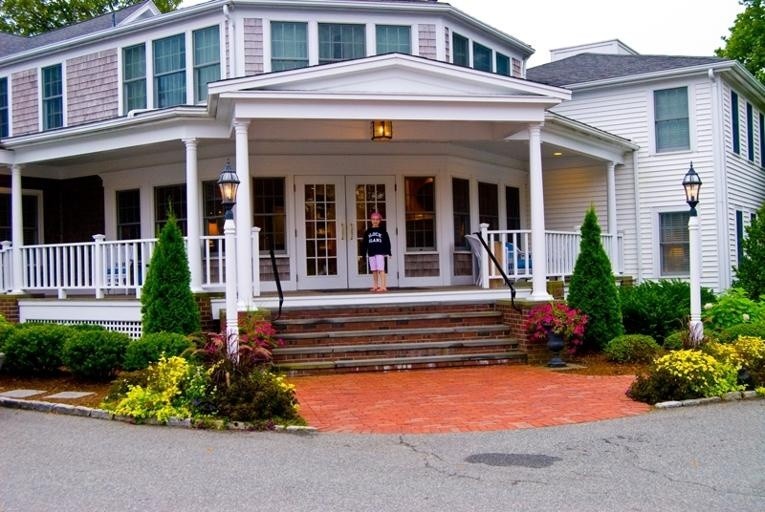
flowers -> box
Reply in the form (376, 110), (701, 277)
(704, 302), (712, 310)
(743, 313), (750, 321)
(206, 314), (287, 361)
(524, 304), (588, 354)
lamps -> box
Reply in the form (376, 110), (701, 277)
(371, 121), (393, 142)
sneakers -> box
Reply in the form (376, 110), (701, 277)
(370, 288), (387, 293)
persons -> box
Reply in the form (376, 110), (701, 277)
(362, 213), (393, 292)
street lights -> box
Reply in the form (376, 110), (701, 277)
(219, 158), (239, 364)
(682, 164), (703, 347)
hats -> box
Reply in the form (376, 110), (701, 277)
(371, 213), (382, 219)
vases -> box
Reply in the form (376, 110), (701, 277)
(545, 331), (566, 368)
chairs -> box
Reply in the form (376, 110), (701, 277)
(502, 240), (535, 288)
